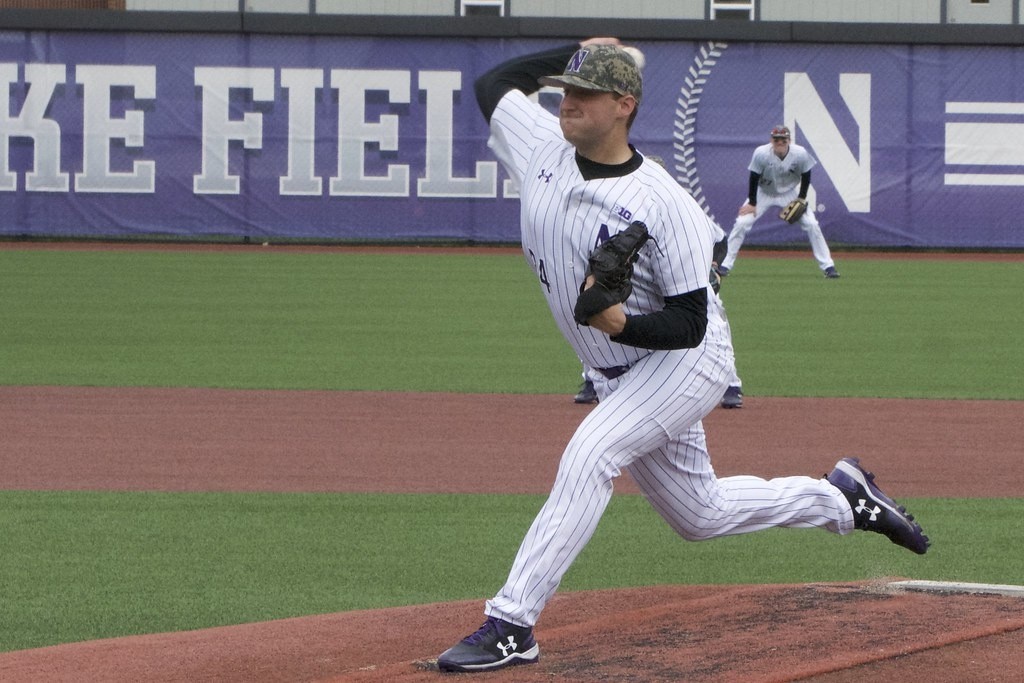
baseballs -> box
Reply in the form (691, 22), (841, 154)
(622, 46), (646, 71)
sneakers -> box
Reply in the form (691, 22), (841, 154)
(573, 381), (599, 403)
(721, 386), (744, 408)
(437, 617), (539, 672)
(822, 456), (931, 555)
(824, 267), (840, 278)
(717, 266), (729, 276)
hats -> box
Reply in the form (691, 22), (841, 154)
(770, 126), (791, 140)
(537, 43), (642, 105)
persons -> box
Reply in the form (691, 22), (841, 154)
(437, 36), (932, 672)
(718, 124), (840, 279)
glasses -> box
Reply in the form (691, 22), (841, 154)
(773, 129), (786, 135)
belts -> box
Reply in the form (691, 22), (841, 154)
(592, 364), (630, 380)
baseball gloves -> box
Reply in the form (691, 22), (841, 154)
(776, 199), (809, 227)
(570, 217), (666, 330)
